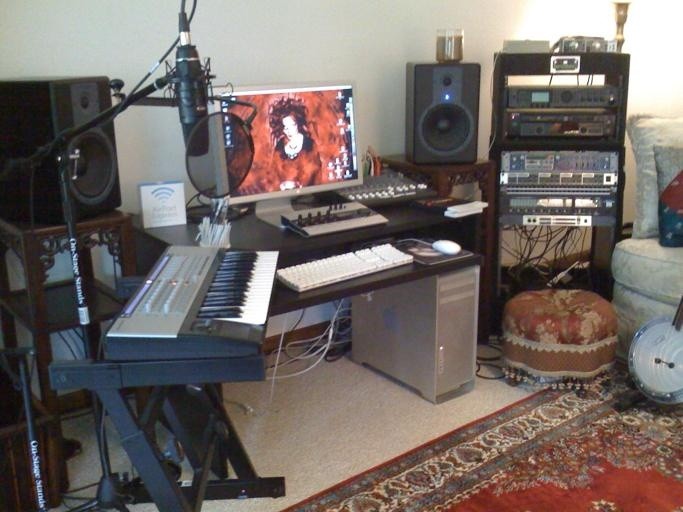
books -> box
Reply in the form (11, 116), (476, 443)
(417, 194), (488, 219)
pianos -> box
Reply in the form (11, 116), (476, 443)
(48, 244), (284, 510)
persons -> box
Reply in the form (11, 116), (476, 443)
(268, 97), (322, 189)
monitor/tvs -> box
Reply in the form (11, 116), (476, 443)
(212, 83), (362, 226)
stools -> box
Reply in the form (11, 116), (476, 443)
(500, 289), (617, 399)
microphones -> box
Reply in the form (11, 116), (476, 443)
(175, 44), (214, 157)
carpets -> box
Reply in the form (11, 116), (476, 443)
(283, 359), (682, 511)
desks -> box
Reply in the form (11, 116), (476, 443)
(0, 204), (132, 511)
(379, 153), (496, 320)
(127, 175), (492, 478)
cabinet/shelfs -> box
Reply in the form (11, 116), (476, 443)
(493, 53), (631, 337)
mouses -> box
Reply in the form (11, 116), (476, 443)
(431, 239), (462, 256)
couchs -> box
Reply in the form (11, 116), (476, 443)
(611, 239), (683, 366)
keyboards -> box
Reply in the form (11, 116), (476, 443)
(278, 242), (413, 292)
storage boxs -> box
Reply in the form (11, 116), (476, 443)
(0, 383), (62, 511)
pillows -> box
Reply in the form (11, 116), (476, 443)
(626, 114), (683, 239)
(653, 145), (683, 248)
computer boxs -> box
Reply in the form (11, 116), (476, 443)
(351, 267), (481, 403)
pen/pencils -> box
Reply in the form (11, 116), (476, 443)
(210, 197), (229, 224)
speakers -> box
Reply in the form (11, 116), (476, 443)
(407, 62), (482, 161)
(1, 76), (123, 224)
